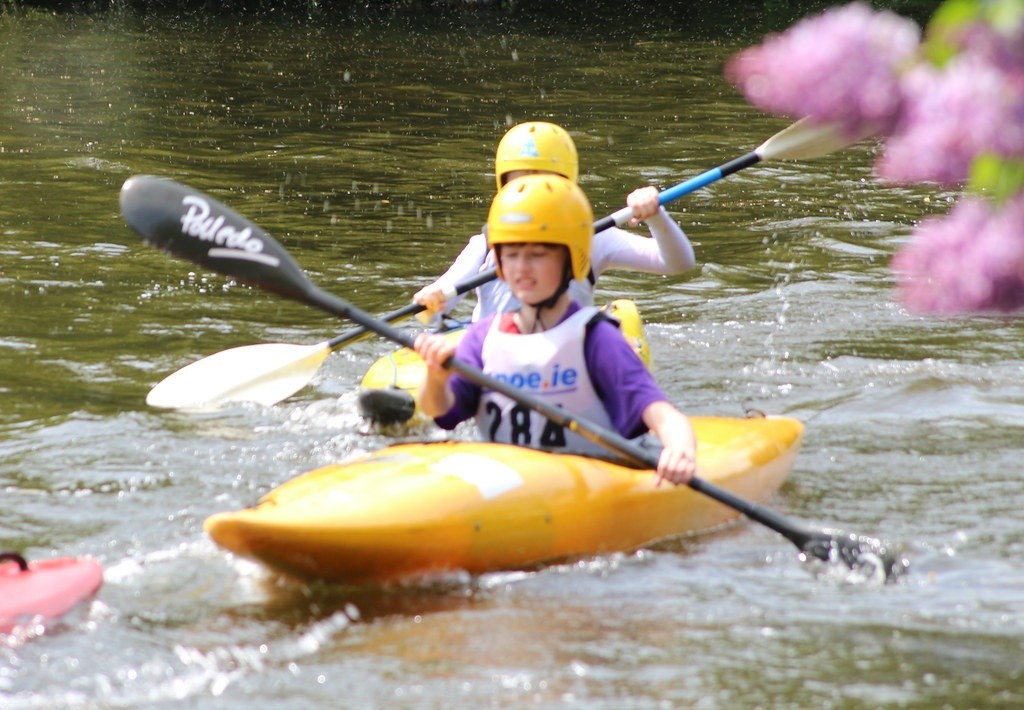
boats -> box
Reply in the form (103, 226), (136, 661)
(358, 298), (653, 438)
(208, 413), (809, 598)
(0, 548), (105, 640)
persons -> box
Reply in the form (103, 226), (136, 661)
(415, 174), (697, 489)
(416, 122), (695, 328)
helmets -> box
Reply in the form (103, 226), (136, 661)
(495, 121), (580, 193)
(487, 174), (595, 282)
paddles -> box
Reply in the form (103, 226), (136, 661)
(145, 111), (879, 410)
(117, 174), (899, 577)
